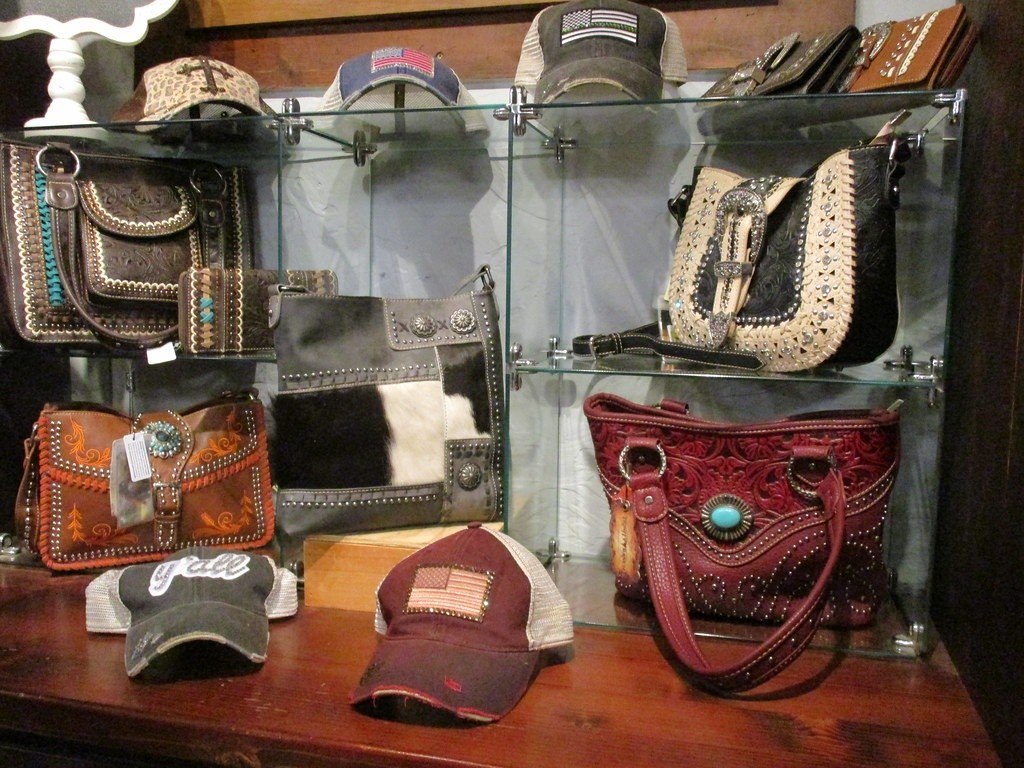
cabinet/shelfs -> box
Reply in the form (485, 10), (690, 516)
(0, 87), (969, 663)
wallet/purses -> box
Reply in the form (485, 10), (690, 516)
(175, 269), (340, 354)
(697, 4), (980, 136)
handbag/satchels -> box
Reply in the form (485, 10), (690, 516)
(573, 109), (932, 365)
(0, 142), (260, 353)
(11, 388), (276, 569)
(267, 264), (505, 538)
(584, 393), (902, 696)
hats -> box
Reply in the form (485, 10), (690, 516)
(514, 0), (689, 106)
(111, 55), (261, 150)
(85, 546), (298, 679)
(351, 520), (576, 722)
(315, 46), (492, 143)
